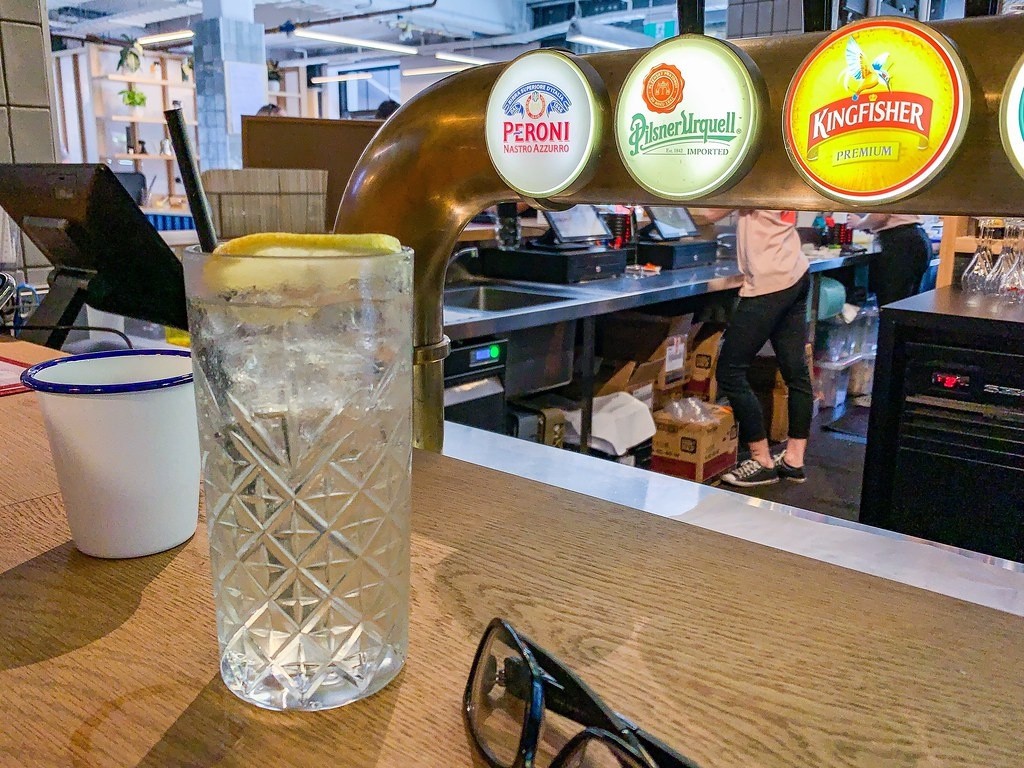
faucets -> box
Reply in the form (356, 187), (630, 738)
(447, 246), (479, 267)
(717, 233), (736, 250)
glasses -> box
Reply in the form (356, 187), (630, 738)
(463, 618), (702, 768)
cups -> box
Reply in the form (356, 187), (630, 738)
(19, 348), (202, 560)
(182, 241), (416, 711)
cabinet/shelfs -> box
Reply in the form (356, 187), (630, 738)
(49, 42), (307, 195)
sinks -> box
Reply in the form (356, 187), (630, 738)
(443, 286), (577, 312)
(715, 257), (737, 261)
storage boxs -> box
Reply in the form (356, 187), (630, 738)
(203, 168), (330, 239)
(558, 309), (878, 483)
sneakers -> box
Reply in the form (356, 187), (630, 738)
(769, 449), (808, 483)
(720, 459), (780, 486)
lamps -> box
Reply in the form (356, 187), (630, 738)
(136, 17), (658, 86)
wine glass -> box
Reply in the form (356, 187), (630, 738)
(960, 216), (1024, 304)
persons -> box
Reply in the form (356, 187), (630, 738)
(847, 212), (933, 310)
(255, 99), (400, 121)
(688, 208), (814, 488)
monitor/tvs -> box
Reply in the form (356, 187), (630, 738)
(542, 205), (614, 244)
(0, 163), (190, 334)
(642, 205), (701, 241)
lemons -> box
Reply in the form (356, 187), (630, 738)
(202, 231), (402, 294)
